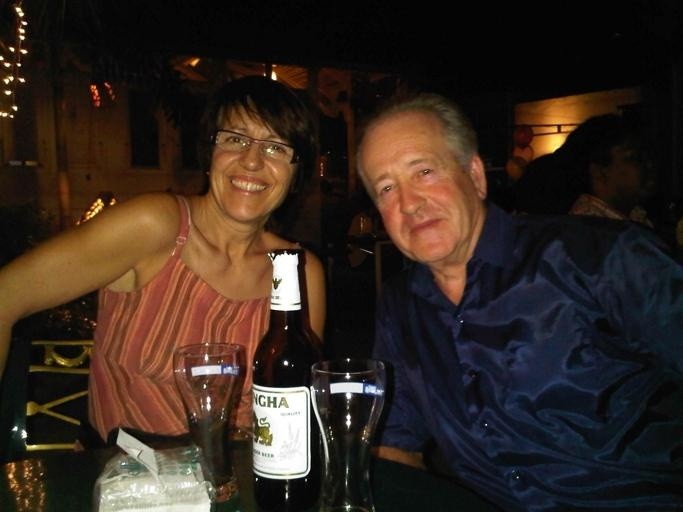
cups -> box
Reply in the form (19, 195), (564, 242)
(311, 360), (386, 512)
(174, 343), (246, 501)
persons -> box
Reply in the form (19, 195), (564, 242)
(351, 86), (683, 512)
(568, 127), (655, 229)
(1, 73), (327, 457)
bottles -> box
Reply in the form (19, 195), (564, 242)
(252, 248), (323, 512)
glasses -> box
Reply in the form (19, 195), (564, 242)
(212, 129), (300, 164)
